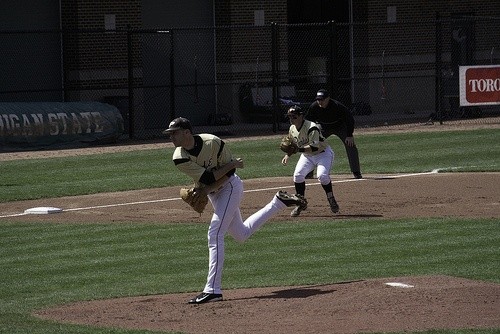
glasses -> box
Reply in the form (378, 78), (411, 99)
(288, 116), (299, 119)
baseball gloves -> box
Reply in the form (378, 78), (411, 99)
(179, 185), (208, 212)
(279, 140), (309, 155)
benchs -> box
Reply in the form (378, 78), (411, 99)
(239, 80), (336, 122)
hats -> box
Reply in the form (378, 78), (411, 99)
(165, 116), (192, 131)
(315, 89), (329, 100)
(285, 105), (302, 116)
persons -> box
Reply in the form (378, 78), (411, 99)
(166, 117), (308, 304)
(304, 89), (362, 179)
(280, 105), (340, 218)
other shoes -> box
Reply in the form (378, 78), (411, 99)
(353, 171), (362, 179)
(305, 174), (313, 178)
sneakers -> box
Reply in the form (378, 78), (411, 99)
(290, 202), (307, 217)
(276, 190), (308, 205)
(328, 195), (339, 213)
(188, 292), (224, 304)
